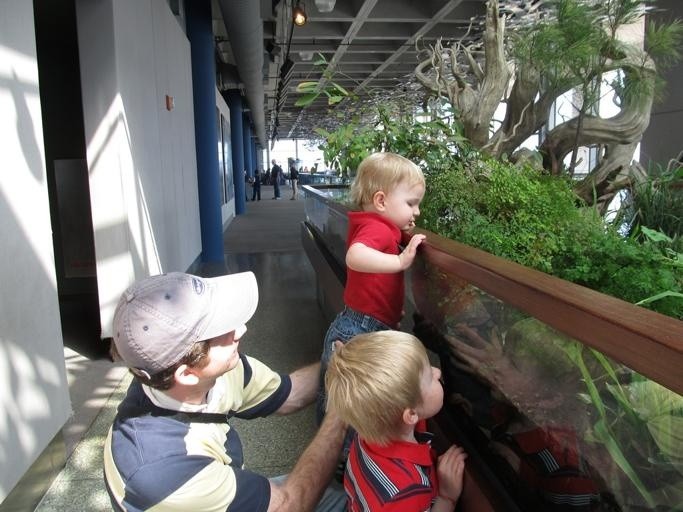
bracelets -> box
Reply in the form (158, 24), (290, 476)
(436, 489), (457, 508)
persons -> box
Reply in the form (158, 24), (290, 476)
(103, 269), (349, 511)
(270, 158), (282, 201)
(316, 151), (427, 486)
(322, 329), (470, 511)
(244, 167), (249, 202)
(250, 168), (261, 202)
(260, 167), (270, 185)
(288, 160), (299, 201)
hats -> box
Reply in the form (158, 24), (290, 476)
(111, 271), (259, 381)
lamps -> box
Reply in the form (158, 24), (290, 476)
(293, 7), (307, 27)
